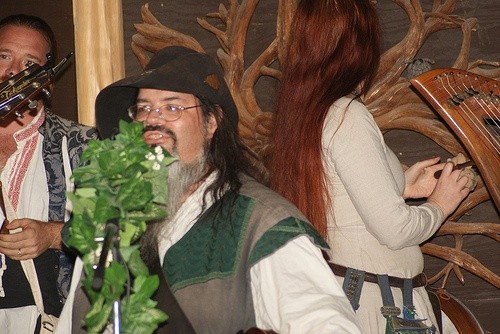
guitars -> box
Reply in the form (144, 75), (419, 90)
(0, 49), (76, 121)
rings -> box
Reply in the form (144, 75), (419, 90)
(18, 248), (22, 257)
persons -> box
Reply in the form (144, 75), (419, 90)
(0, 14), (97, 334)
(267, 1), (478, 334)
(54, 45), (365, 334)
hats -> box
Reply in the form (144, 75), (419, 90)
(94, 45), (238, 149)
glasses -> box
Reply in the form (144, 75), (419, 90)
(124, 102), (206, 122)
